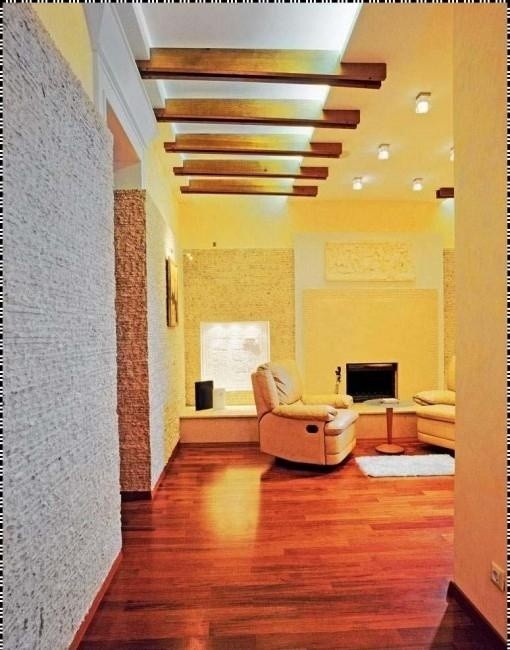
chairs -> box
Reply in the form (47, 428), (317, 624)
(413, 355), (456, 451)
(251, 359), (359, 467)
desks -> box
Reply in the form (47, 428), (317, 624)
(180, 399), (418, 442)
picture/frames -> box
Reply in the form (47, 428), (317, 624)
(167, 257), (181, 328)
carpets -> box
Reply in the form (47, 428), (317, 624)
(354, 454), (456, 477)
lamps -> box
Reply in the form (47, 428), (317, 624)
(414, 91), (432, 113)
(350, 176), (363, 192)
(412, 177), (424, 191)
(377, 142), (389, 160)
